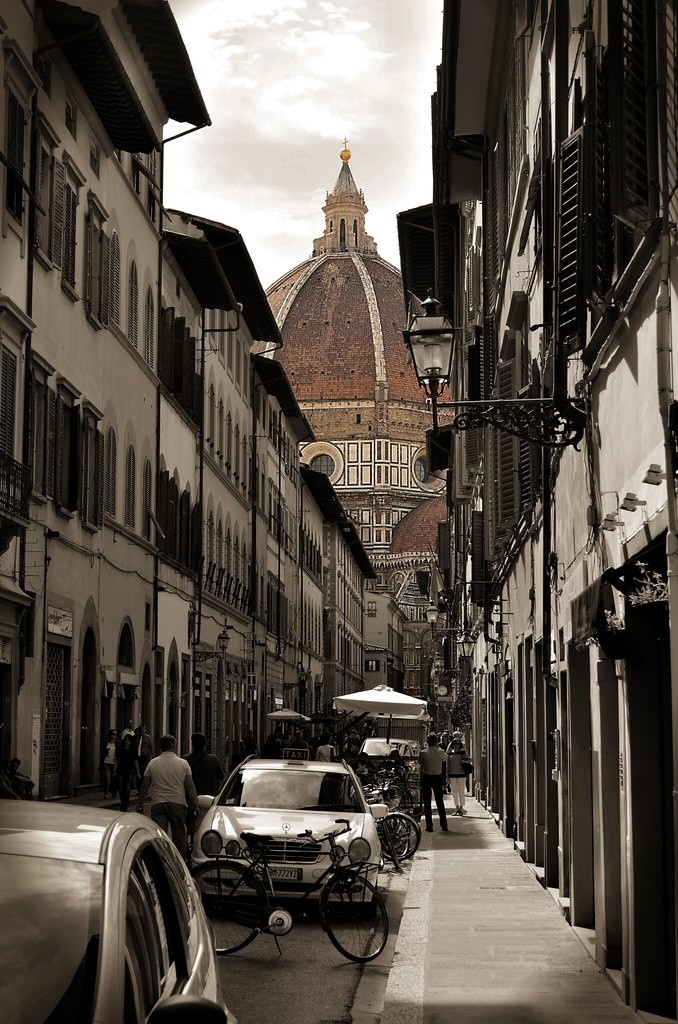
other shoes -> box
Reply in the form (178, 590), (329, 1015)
(443, 789), (447, 794)
(447, 786), (452, 792)
(452, 810), (458, 816)
(425, 828), (433, 832)
(459, 811), (463, 816)
(104, 794), (108, 800)
(442, 827), (448, 831)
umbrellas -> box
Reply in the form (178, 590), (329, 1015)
(267, 708), (311, 734)
(333, 684), (429, 744)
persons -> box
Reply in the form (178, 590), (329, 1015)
(137, 734), (198, 858)
(294, 730), (309, 750)
(102, 719), (155, 811)
(418, 733), (448, 832)
(350, 730), (367, 752)
(357, 752), (376, 770)
(437, 730), (462, 794)
(447, 739), (472, 816)
(315, 733), (336, 762)
(243, 730), (256, 756)
(0, 758), (35, 800)
(389, 748), (408, 773)
(181, 733), (224, 831)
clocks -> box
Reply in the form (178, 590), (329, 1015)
(437, 685), (448, 696)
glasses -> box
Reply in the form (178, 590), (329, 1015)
(113, 733), (117, 735)
(443, 736), (447, 738)
(128, 723), (133, 724)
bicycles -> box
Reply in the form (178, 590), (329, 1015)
(350, 759), (425, 870)
(190, 818), (391, 964)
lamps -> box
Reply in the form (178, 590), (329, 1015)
(599, 464), (669, 544)
(284, 667), (309, 690)
(196, 628), (231, 663)
(403, 287), (588, 452)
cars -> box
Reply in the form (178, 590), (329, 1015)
(190, 751), (392, 920)
(356, 736), (425, 773)
(0, 795), (238, 1024)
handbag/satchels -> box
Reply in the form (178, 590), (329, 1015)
(461, 751), (474, 774)
